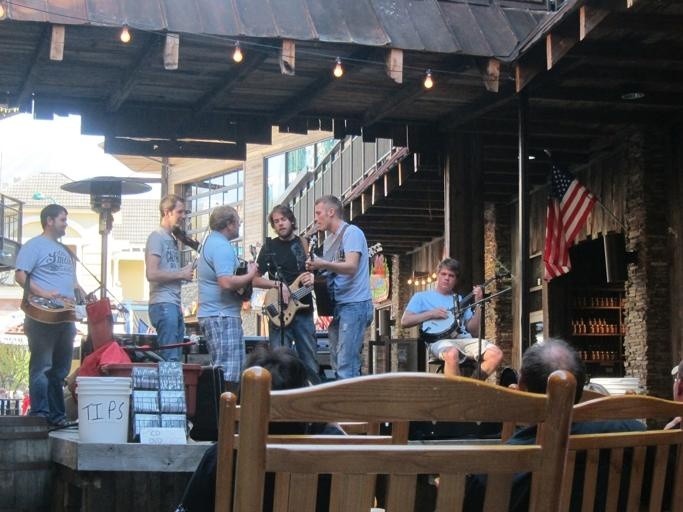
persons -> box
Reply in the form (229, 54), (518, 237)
(196, 205), (259, 385)
(303, 194), (375, 379)
(12, 203), (97, 428)
(460, 337), (658, 511)
(170, 340), (348, 511)
(142, 193), (195, 366)
(252, 203), (323, 386)
(400, 257), (504, 382)
(656, 358), (682, 512)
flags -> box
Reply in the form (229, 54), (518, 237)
(540, 157), (598, 284)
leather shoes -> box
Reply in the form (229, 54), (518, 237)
(57, 420), (71, 427)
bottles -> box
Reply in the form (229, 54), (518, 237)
(566, 296), (626, 360)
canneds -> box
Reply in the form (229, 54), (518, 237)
(569, 296), (618, 361)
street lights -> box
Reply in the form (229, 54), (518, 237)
(32, 191), (63, 245)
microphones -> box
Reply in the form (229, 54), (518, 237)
(74, 287), (81, 305)
(265, 236), (272, 262)
(452, 293), (462, 326)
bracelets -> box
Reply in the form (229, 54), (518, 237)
(273, 280), (280, 288)
(476, 302), (483, 306)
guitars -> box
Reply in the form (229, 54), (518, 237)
(264, 242), (382, 327)
(310, 235), (336, 317)
(21, 293), (130, 325)
(231, 245), (257, 300)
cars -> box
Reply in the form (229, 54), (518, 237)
(0, 309), (89, 350)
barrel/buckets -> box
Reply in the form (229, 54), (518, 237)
(2, 414), (52, 512)
(590, 376), (641, 398)
(73, 375), (132, 445)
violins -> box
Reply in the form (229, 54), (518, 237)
(173, 227), (203, 253)
